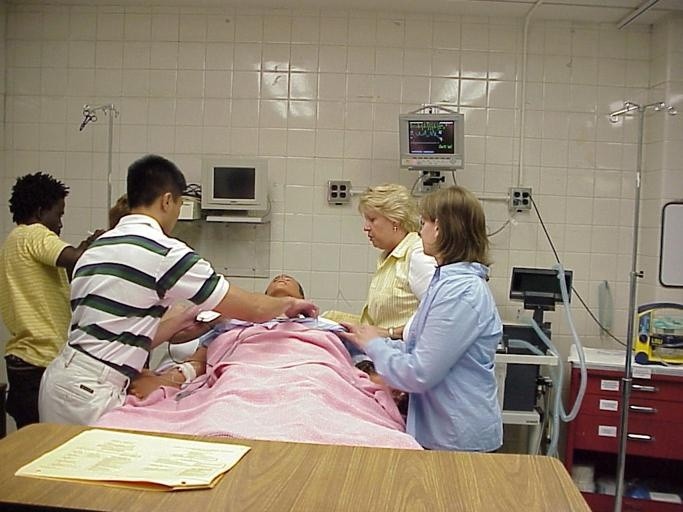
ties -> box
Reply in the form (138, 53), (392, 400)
(200, 157), (269, 211)
(397, 111), (465, 172)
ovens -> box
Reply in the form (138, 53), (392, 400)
(151, 295), (415, 485)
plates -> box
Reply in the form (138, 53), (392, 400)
(566, 344), (683, 491)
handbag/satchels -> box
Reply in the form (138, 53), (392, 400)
(389, 327), (393, 337)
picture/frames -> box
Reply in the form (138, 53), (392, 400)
(1, 421), (597, 511)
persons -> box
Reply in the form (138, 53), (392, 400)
(334, 186), (504, 452)
(0, 171), (105, 430)
(130, 274), (407, 406)
(38, 155), (320, 426)
(109, 193), (200, 351)
(358, 184), (438, 338)
